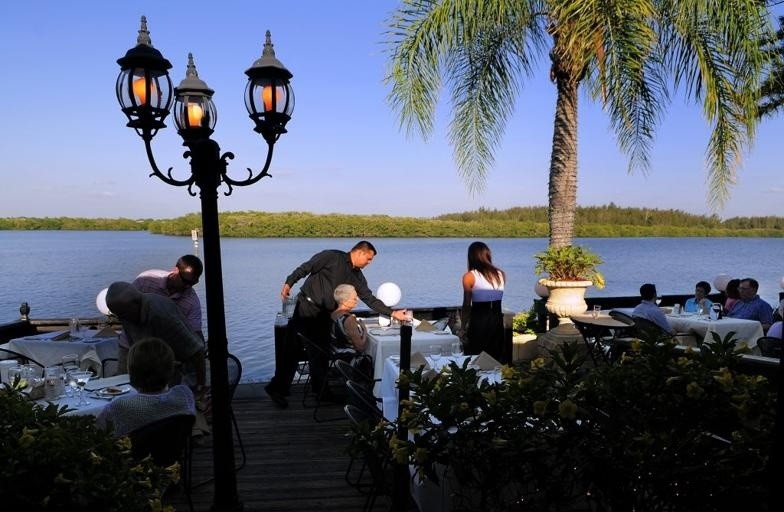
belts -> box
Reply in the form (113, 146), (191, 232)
(300, 290), (317, 307)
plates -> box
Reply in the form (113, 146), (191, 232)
(671, 315), (680, 317)
(100, 386), (130, 395)
(681, 313), (692, 316)
(432, 331), (450, 335)
(82, 337), (102, 342)
(390, 356), (400, 360)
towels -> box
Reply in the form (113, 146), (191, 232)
(93, 326), (120, 338)
(396, 352), (430, 371)
(415, 319), (439, 332)
(469, 351), (503, 370)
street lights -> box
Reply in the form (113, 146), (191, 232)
(115, 16), (295, 512)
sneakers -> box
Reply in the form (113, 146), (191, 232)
(192, 435), (206, 453)
(264, 382), (288, 409)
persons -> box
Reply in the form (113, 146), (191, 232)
(462, 242), (506, 355)
(96, 338), (196, 443)
(767, 297), (784, 337)
(329, 284), (368, 369)
(724, 279), (743, 311)
(114, 255), (206, 378)
(685, 281), (713, 314)
(264, 241), (413, 410)
(727, 278), (773, 330)
(631, 284), (677, 338)
(106, 282), (204, 390)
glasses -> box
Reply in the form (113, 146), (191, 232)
(738, 287), (751, 290)
(178, 271), (199, 285)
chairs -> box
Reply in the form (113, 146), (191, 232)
(345, 405), (411, 512)
(191, 354), (247, 491)
(296, 361), (320, 397)
(345, 380), (395, 496)
(757, 337), (783, 358)
(128, 412), (198, 511)
(633, 315), (672, 345)
(297, 332), (367, 422)
(335, 358), (374, 414)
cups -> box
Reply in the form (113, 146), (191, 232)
(62, 354), (79, 371)
(593, 305), (601, 319)
(45, 366), (60, 380)
(70, 371), (93, 406)
(24, 364), (36, 378)
(451, 342), (464, 362)
(430, 345), (441, 368)
(9, 367), (21, 380)
